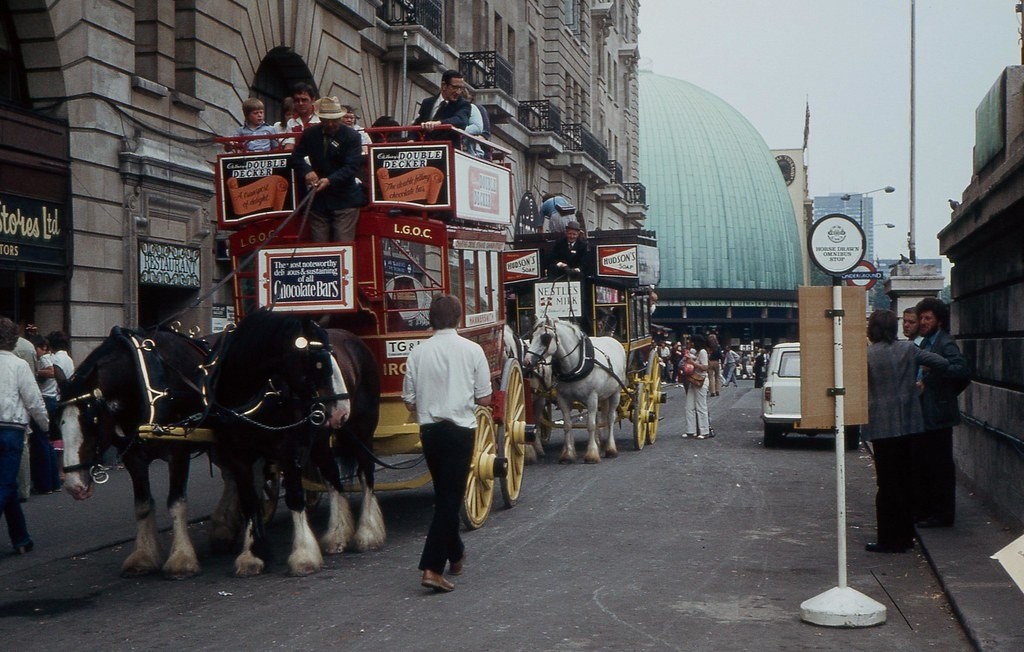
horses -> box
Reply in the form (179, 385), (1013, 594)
(504, 313), (627, 464)
(210, 306), (386, 576)
(54, 326), (239, 582)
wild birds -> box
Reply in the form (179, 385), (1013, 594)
(947, 198), (959, 210)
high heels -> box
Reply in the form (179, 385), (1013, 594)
(15, 540), (33, 554)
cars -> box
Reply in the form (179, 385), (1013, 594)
(761, 342), (860, 450)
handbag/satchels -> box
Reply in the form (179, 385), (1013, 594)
(555, 203), (575, 215)
(736, 362), (739, 367)
(688, 372), (705, 388)
(753, 366), (756, 372)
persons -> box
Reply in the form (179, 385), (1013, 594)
(283, 81), (322, 150)
(537, 193), (578, 234)
(903, 307), (925, 348)
(11, 321), (75, 502)
(273, 96), (295, 148)
(340, 105), (372, 154)
(290, 96), (363, 327)
(0, 317), (50, 553)
(865, 309), (950, 554)
(223, 98), (280, 152)
(649, 292), (657, 315)
(913, 298), (972, 528)
(680, 335), (710, 439)
(407, 69), (493, 163)
(655, 334), (770, 397)
(401, 294), (493, 592)
(547, 221), (588, 282)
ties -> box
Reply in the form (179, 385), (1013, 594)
(432, 101), (446, 121)
(568, 243), (572, 249)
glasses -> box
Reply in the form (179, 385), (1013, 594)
(446, 83), (465, 90)
(292, 98), (312, 102)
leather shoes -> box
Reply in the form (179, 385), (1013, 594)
(421, 570), (455, 592)
(449, 556), (463, 575)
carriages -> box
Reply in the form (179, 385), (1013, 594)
(504, 228), (668, 465)
(53, 124), (539, 579)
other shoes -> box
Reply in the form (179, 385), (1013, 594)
(53, 488), (61, 492)
(20, 497), (28, 503)
(33, 489), (52, 494)
(682, 432), (696, 438)
(866, 542), (906, 553)
(906, 540), (914, 549)
(660, 379), (684, 388)
(917, 515), (954, 529)
(709, 384), (737, 397)
(696, 435), (709, 439)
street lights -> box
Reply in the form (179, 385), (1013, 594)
(840, 186), (896, 231)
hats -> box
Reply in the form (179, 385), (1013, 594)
(565, 221), (583, 231)
(313, 96), (348, 119)
